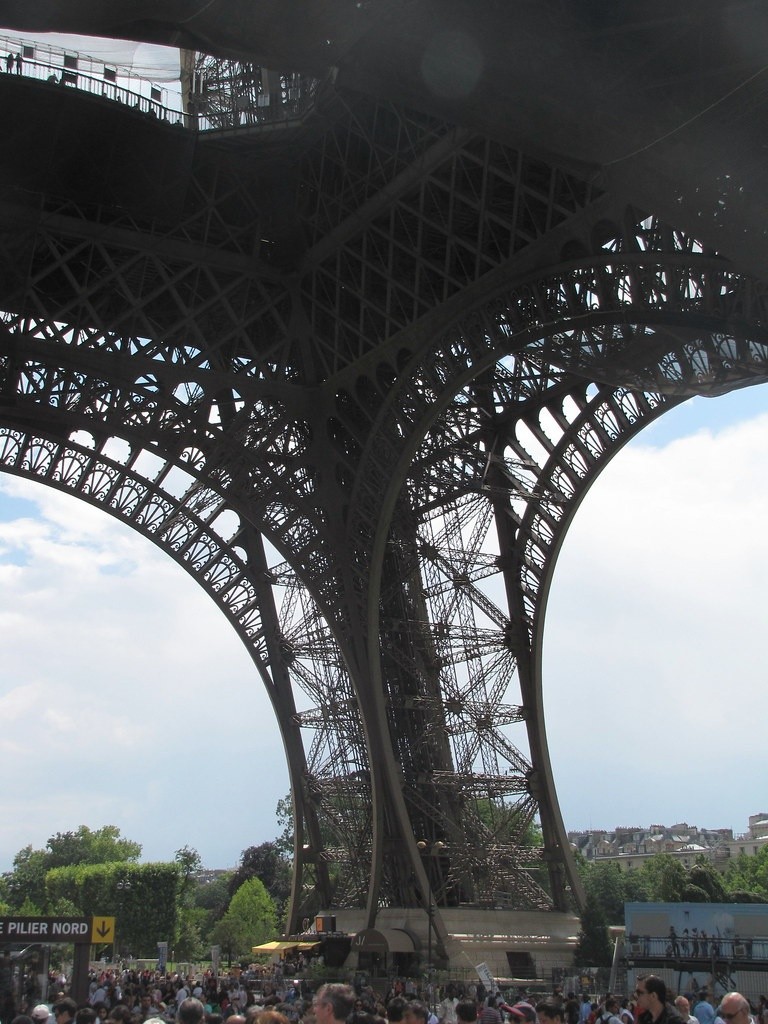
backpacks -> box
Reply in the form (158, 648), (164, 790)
(600, 1015), (613, 1024)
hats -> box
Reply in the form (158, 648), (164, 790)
(32, 1005), (52, 1019)
(58, 992), (64, 995)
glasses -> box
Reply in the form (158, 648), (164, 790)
(720, 1009), (742, 1019)
(142, 998), (151, 1001)
(636, 989), (648, 996)
(312, 996), (326, 1005)
(55, 1013), (61, 1018)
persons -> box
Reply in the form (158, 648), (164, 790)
(633, 973), (693, 1023)
(272, 945), (321, 972)
(721, 992), (758, 1023)
(6, 50), (182, 130)
(666, 927), (754, 961)
(11, 970), (768, 1024)
(313, 981), (356, 1024)
